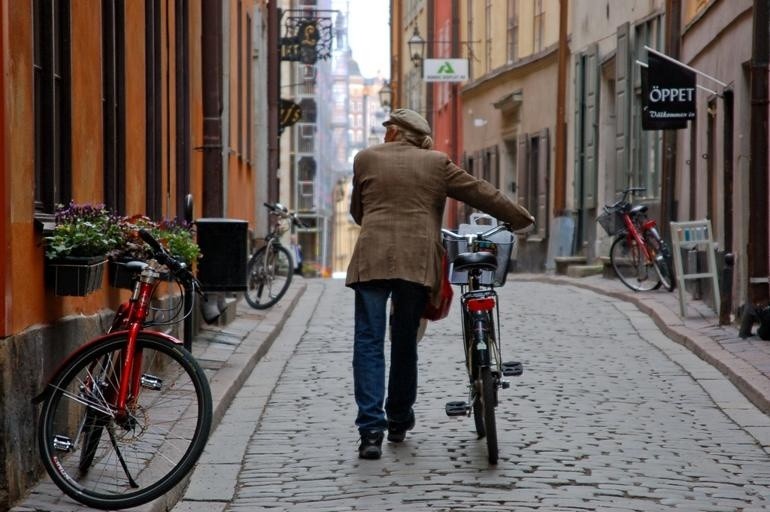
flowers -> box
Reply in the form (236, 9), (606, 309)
(105, 214), (160, 263)
(158, 215), (204, 265)
(43, 197), (124, 259)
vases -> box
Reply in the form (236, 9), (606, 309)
(48, 256), (110, 297)
(108, 256), (146, 290)
(154, 255), (193, 282)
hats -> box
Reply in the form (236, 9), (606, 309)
(382, 108), (431, 136)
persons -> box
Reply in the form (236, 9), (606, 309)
(291, 234), (304, 277)
(346, 108), (534, 460)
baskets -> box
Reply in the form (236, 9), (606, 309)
(440, 224), (518, 287)
(597, 205), (628, 237)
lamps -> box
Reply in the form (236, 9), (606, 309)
(407, 24), (481, 69)
(378, 79), (428, 115)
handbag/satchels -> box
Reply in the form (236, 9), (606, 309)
(445, 212), (512, 289)
(419, 238), (453, 325)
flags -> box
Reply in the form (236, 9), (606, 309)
(639, 66), (688, 133)
(646, 52), (699, 121)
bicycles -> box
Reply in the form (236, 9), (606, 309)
(243, 202), (308, 310)
(440, 218), (534, 463)
(30, 228), (212, 512)
(599, 172), (677, 293)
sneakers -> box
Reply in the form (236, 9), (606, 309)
(387, 421), (407, 444)
(356, 429), (383, 460)
